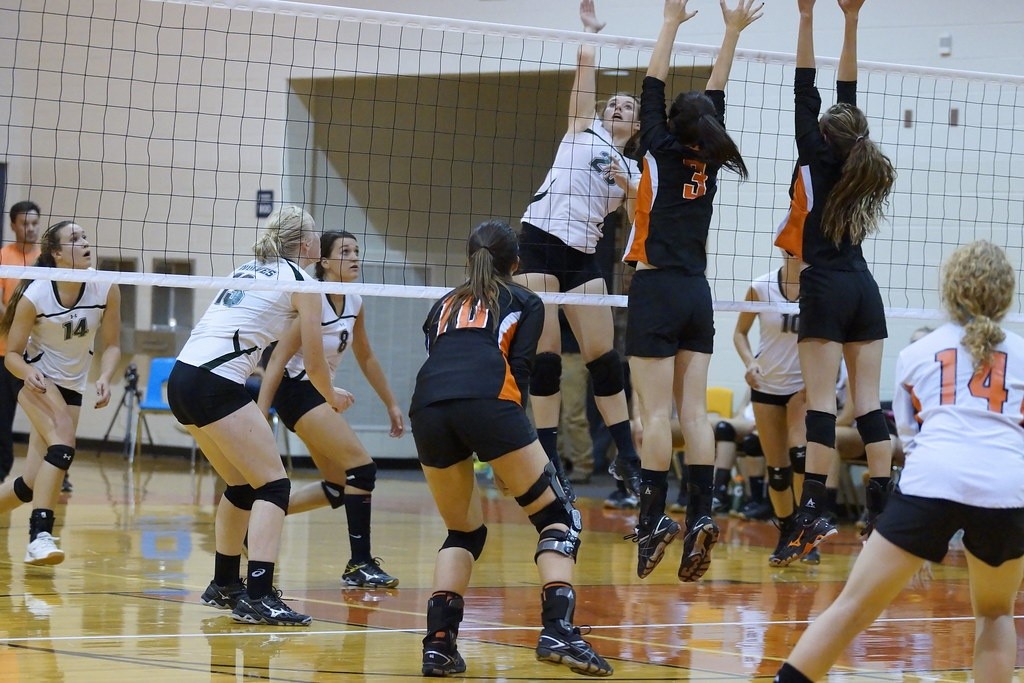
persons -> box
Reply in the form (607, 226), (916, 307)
(616, 327), (936, 530)
(406, 221), (615, 675)
(168, 205), (354, 625)
(0, 201), (71, 493)
(0, 220), (122, 565)
(734, 219), (820, 564)
(587, 336), (640, 508)
(518, 0), (642, 486)
(773, 0), (895, 531)
(771, 239), (1024, 683)
(260, 229), (404, 594)
(625, 0), (766, 581)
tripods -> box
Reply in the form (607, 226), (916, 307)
(97, 381), (155, 460)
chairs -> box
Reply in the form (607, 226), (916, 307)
(247, 376), (292, 470)
(128, 357), (197, 474)
(671, 388), (733, 480)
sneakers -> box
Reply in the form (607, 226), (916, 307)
(677, 516), (720, 582)
(608, 455), (643, 495)
(230, 584), (312, 627)
(341, 556), (399, 588)
(199, 576), (266, 624)
(768, 515), (801, 561)
(623, 514), (682, 579)
(24, 531), (65, 567)
(772, 515), (839, 566)
(800, 548), (820, 565)
(621, 495), (642, 510)
(536, 623), (613, 677)
(422, 640), (466, 676)
(603, 489), (627, 508)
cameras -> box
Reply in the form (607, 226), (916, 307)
(125, 365), (139, 381)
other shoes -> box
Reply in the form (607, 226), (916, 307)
(710, 494), (731, 512)
(729, 500), (772, 521)
(670, 493), (687, 513)
